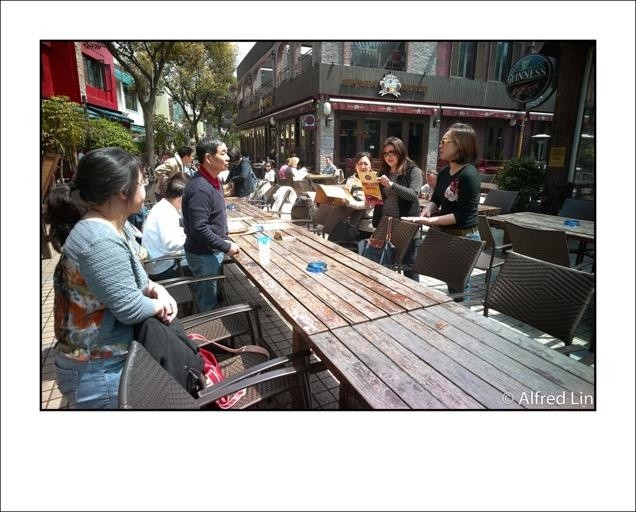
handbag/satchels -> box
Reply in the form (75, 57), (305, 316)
(221, 177), (235, 197)
(362, 215), (396, 268)
(188, 329), (272, 411)
(328, 214), (359, 246)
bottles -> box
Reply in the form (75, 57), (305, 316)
(257, 237), (272, 266)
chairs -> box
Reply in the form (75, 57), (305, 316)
(114, 254), (313, 410)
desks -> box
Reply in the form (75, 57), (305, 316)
(305, 301), (595, 409)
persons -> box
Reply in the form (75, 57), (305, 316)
(52, 120), (483, 411)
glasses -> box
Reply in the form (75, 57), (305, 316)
(382, 149), (398, 158)
(137, 177), (150, 187)
(438, 139), (454, 144)
